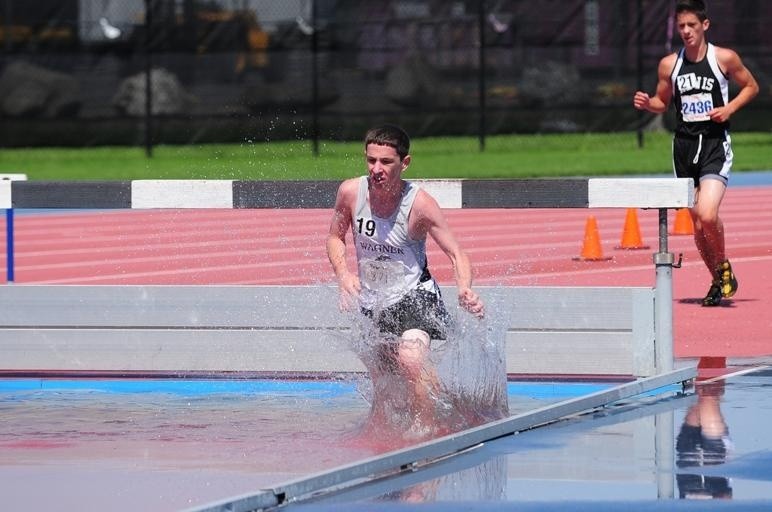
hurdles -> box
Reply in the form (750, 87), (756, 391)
(0, 173), (696, 383)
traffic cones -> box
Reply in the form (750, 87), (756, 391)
(616, 208), (651, 250)
(671, 208), (693, 235)
(572, 213), (610, 262)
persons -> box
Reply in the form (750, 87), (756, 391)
(326, 123), (486, 412)
(630, 1), (761, 306)
(674, 356), (733, 501)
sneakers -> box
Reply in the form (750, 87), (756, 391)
(703, 281), (722, 306)
(718, 261), (739, 298)
